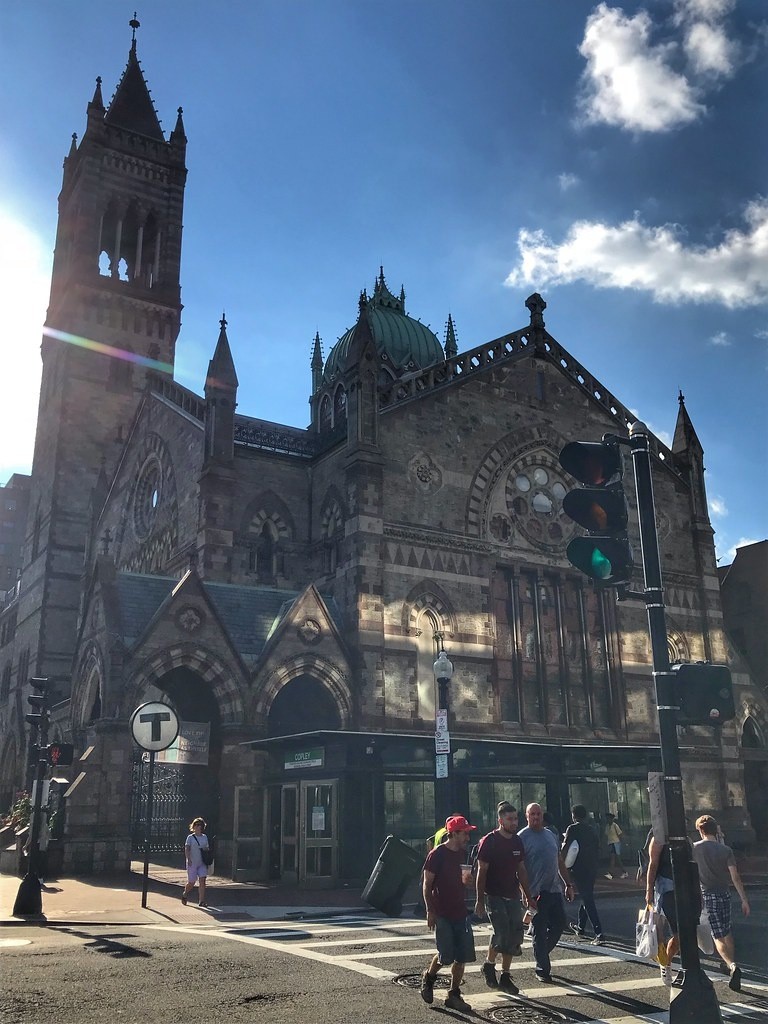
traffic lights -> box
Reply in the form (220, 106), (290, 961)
(47, 743), (74, 766)
(26, 676), (49, 727)
(558, 440), (632, 585)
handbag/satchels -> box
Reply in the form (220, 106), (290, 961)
(561, 832), (579, 868)
(635, 902), (658, 959)
(696, 924), (715, 955)
(200, 847), (214, 865)
(638, 909), (665, 944)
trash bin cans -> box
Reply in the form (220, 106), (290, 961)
(359, 833), (427, 917)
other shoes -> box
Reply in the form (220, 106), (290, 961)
(535, 973), (552, 984)
(480, 963), (499, 988)
(728, 965), (742, 993)
(199, 902), (207, 907)
(591, 933), (605, 945)
(182, 891), (187, 905)
(497, 972), (519, 995)
(619, 872), (629, 879)
(604, 872), (612, 880)
(720, 962), (731, 976)
(414, 903), (426, 918)
(569, 922), (584, 936)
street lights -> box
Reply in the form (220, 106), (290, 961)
(433, 654), (453, 821)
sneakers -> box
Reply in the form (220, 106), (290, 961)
(421, 969), (437, 1004)
(444, 987), (471, 1012)
(659, 962), (673, 987)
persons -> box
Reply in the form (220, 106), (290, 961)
(420, 816), (477, 1011)
(426, 800), (575, 936)
(474, 804), (537, 994)
(181, 818), (210, 908)
(602, 812), (629, 880)
(637, 814), (704, 987)
(561, 804), (605, 946)
(517, 802), (575, 982)
(693, 814), (751, 991)
(22, 811), (50, 888)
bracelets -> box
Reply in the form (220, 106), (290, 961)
(565, 884), (576, 888)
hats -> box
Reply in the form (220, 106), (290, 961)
(447, 816), (477, 832)
